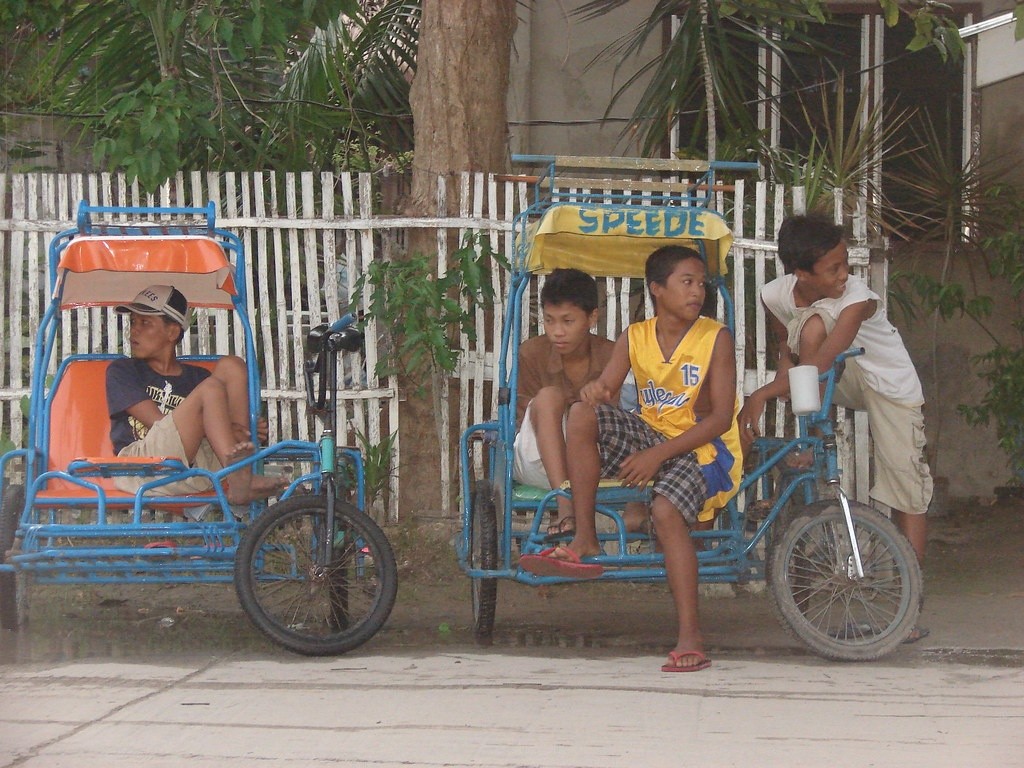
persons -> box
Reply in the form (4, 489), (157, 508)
(106, 285), (289, 506)
(515, 246), (743, 673)
(737, 215), (936, 642)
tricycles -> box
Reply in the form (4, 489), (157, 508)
(0, 199), (399, 658)
(452, 152), (926, 664)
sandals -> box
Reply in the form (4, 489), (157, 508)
(902, 625), (930, 644)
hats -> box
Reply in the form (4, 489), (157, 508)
(113, 285), (192, 333)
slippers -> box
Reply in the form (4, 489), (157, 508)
(621, 519), (658, 544)
(542, 517), (578, 541)
(518, 546), (605, 579)
(661, 650), (712, 672)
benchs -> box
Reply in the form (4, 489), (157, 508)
(510, 382), (670, 510)
(33, 353), (249, 508)
